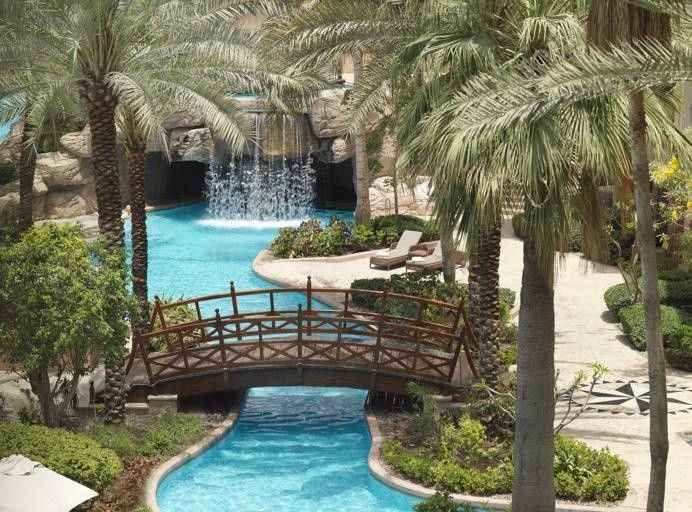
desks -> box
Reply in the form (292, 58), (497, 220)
(409, 244), (435, 259)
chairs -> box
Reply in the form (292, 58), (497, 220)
(407, 236), (465, 273)
(369, 230), (423, 270)
